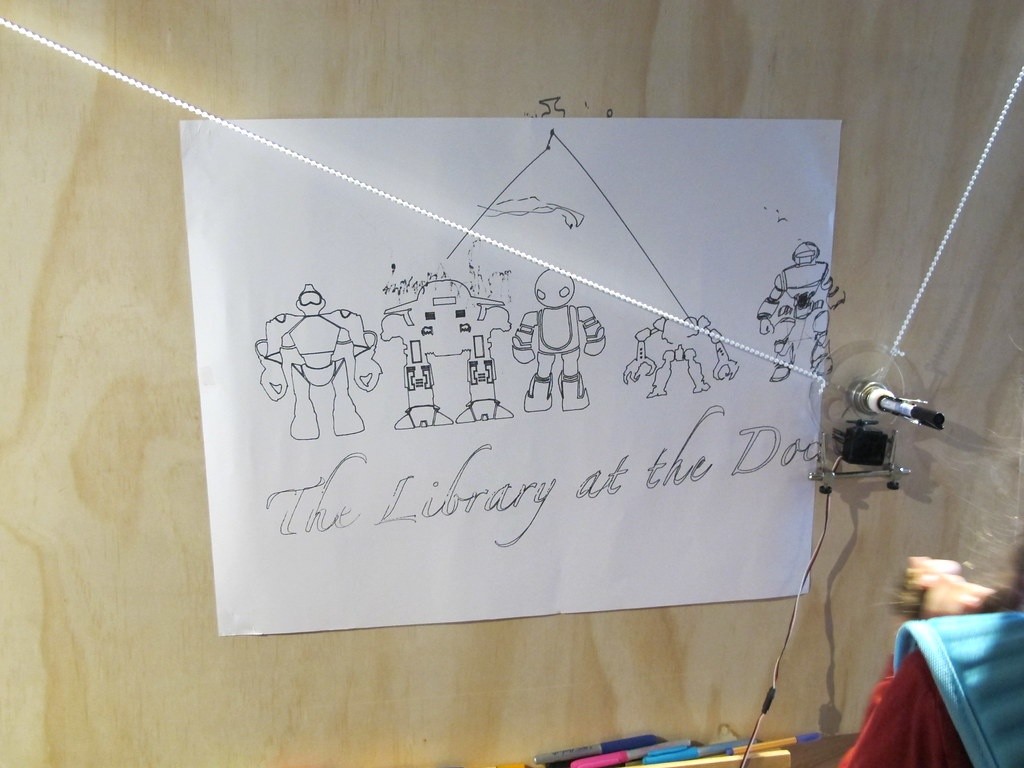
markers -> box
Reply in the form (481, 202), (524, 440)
(727, 732), (820, 756)
(569, 737), (690, 767)
(532, 734), (657, 764)
(642, 735), (758, 765)
